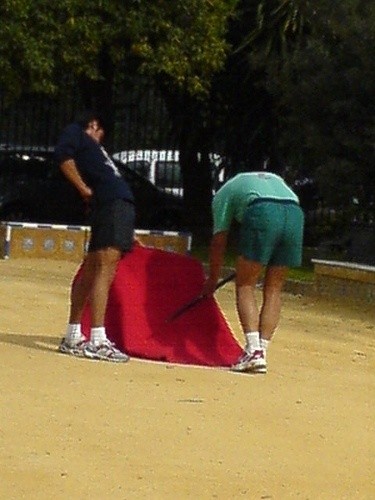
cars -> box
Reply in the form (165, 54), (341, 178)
(0, 145), (184, 231)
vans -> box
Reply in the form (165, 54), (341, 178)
(113, 150), (225, 197)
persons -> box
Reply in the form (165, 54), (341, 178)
(48, 116), (136, 362)
(203, 171), (304, 373)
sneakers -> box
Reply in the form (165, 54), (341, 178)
(58, 333), (89, 357)
(82, 338), (128, 362)
(230, 349), (266, 371)
